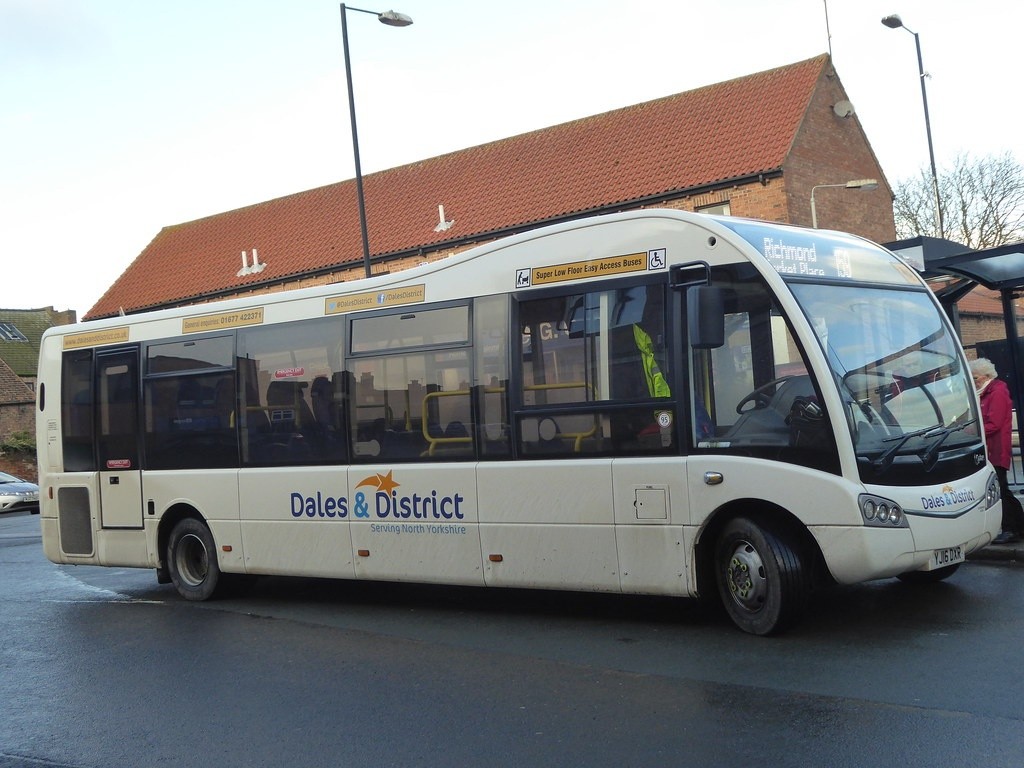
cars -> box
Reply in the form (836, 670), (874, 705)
(0, 471), (40, 518)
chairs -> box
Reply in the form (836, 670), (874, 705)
(139, 414), (565, 470)
(663, 378), (715, 443)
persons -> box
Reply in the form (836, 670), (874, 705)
(964, 358), (1024, 543)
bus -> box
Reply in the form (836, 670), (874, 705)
(36, 208), (1004, 639)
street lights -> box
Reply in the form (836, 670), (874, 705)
(879, 12), (945, 241)
(811, 179), (879, 229)
(341, 1), (415, 278)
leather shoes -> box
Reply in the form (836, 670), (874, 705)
(991, 530), (1021, 544)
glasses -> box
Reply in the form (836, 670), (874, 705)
(974, 375), (986, 382)
